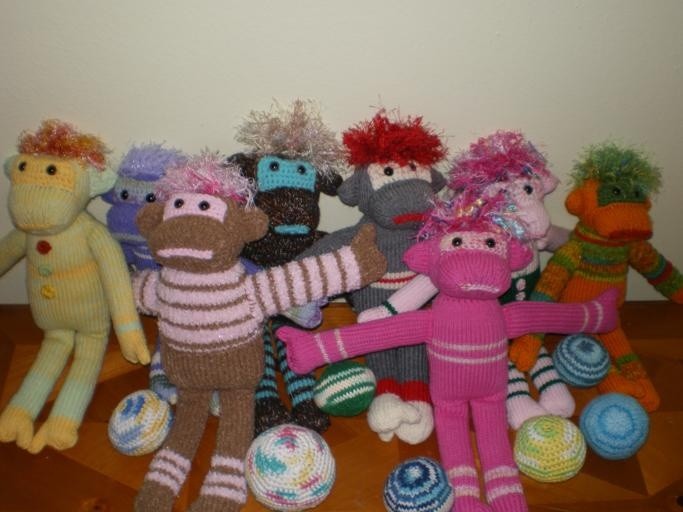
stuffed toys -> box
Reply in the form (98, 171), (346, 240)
(0, 99), (683, 512)
